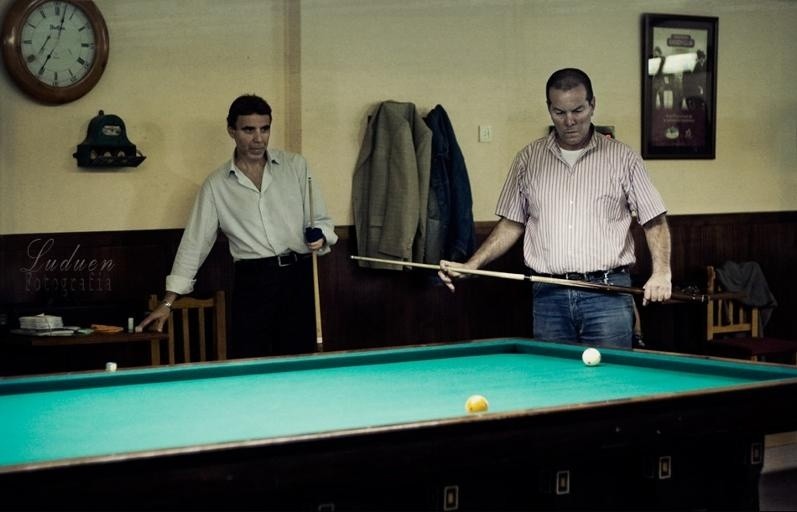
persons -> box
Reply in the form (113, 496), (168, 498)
(134, 93), (339, 357)
(437, 68), (672, 352)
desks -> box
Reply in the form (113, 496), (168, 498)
(0, 338), (797, 512)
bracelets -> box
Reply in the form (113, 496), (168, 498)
(160, 300), (174, 312)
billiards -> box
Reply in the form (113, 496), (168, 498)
(465, 394), (487, 413)
(582, 346), (601, 366)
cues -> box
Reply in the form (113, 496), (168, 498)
(307, 177), (323, 353)
(350, 255), (703, 305)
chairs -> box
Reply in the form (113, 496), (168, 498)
(147, 289), (229, 366)
(702, 262), (761, 361)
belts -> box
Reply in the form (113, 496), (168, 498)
(235, 253), (312, 269)
(535, 265), (630, 281)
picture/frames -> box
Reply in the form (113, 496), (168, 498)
(638, 8), (719, 163)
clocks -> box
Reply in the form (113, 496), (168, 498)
(1, 0), (109, 109)
